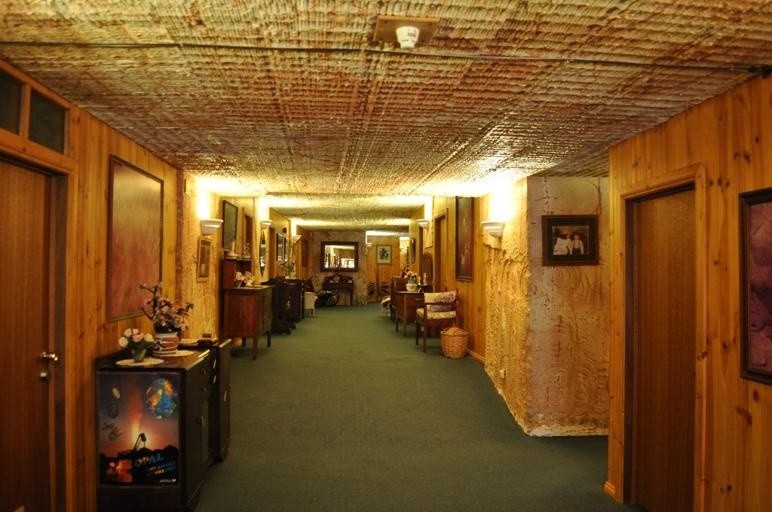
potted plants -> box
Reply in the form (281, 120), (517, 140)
(280, 263), (295, 280)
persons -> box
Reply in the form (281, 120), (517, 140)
(569, 231), (585, 257)
(554, 228), (571, 257)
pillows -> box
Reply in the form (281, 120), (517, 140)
(424, 291), (457, 312)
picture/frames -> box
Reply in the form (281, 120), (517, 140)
(455, 195), (474, 283)
(222, 200), (238, 250)
(197, 237), (212, 282)
(737, 185), (772, 386)
(376, 244), (392, 265)
(104, 151), (166, 326)
(410, 239), (416, 263)
(276, 233), (289, 263)
(541, 214), (599, 267)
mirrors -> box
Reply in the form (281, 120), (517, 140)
(320, 242), (358, 272)
(260, 231), (267, 276)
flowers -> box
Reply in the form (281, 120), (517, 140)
(404, 271), (417, 281)
(118, 328), (156, 350)
(136, 282), (195, 332)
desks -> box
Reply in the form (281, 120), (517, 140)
(394, 291), (425, 336)
(220, 284), (276, 360)
(391, 276), (409, 319)
(323, 274), (353, 307)
(261, 278), (305, 335)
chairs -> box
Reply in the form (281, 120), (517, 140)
(416, 286), (459, 352)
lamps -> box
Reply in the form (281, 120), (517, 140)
(292, 235), (301, 244)
(416, 219), (430, 228)
(481, 219), (505, 239)
(260, 220), (272, 228)
(200, 218), (224, 235)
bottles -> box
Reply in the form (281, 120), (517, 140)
(421, 273), (429, 285)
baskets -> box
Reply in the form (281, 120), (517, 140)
(439, 328), (469, 358)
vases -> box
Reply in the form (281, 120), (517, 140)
(406, 281), (417, 291)
(131, 349), (147, 361)
(153, 332), (181, 353)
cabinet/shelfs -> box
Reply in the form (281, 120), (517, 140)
(97, 337), (233, 510)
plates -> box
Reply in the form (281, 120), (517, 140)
(179, 338), (205, 345)
(114, 357), (164, 366)
